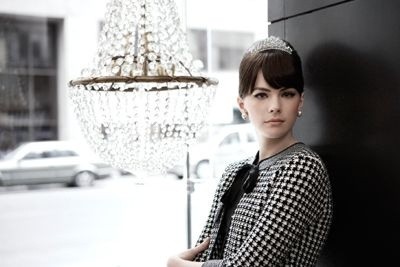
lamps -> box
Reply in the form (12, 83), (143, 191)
(64, 0), (222, 180)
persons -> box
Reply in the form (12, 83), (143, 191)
(166, 34), (336, 267)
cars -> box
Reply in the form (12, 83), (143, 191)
(164, 123), (262, 179)
(1, 140), (109, 190)
